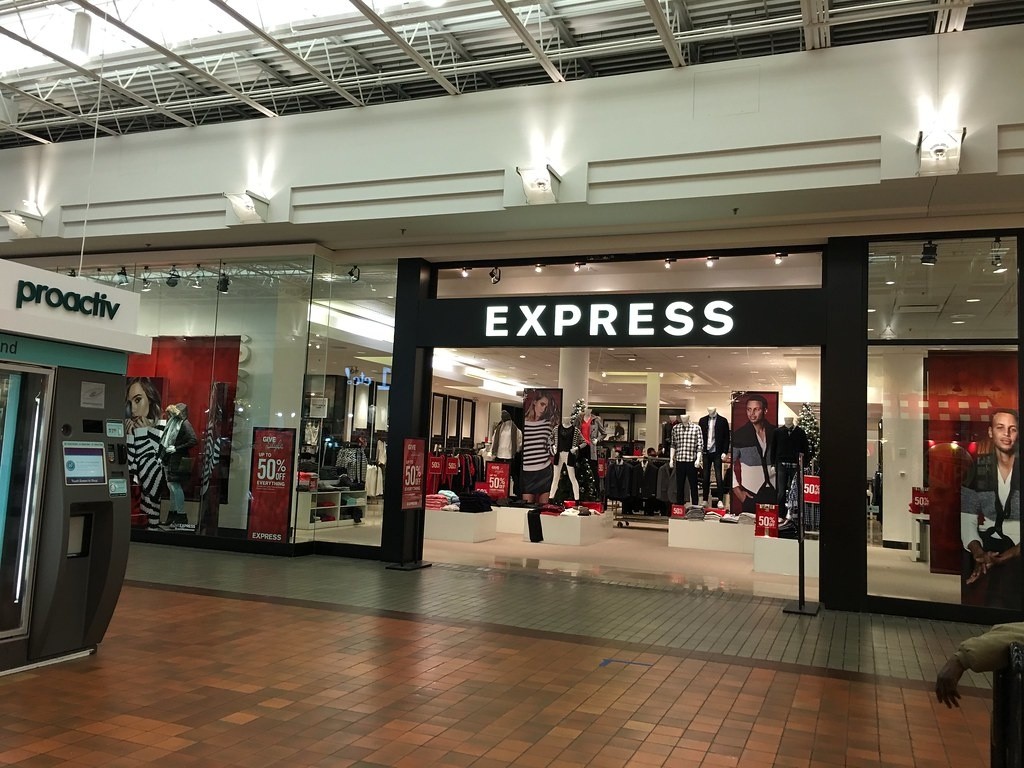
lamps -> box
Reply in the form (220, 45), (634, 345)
(222, 190), (269, 223)
(167, 264), (180, 288)
(920, 239), (938, 266)
(995, 256), (1002, 267)
(192, 278), (201, 289)
(915, 127), (967, 176)
(348, 266), (360, 283)
(217, 273), (229, 292)
(117, 267), (129, 285)
(489, 266), (501, 284)
(0, 202), (43, 241)
(517, 164), (562, 204)
(142, 279), (151, 292)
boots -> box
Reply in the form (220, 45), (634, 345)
(164, 510), (177, 526)
(173, 513), (188, 524)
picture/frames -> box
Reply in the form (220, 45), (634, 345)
(603, 419), (629, 442)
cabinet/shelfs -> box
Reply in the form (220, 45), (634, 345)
(296, 490), (367, 530)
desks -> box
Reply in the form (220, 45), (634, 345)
(908, 512), (930, 561)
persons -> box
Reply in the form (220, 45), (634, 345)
(732, 395), (777, 513)
(643, 448), (656, 474)
(549, 417), (587, 506)
(158, 403), (197, 528)
(699, 407), (730, 508)
(524, 391), (560, 504)
(936, 622), (1024, 709)
(960, 408), (1020, 602)
(575, 408), (607, 501)
(608, 421), (624, 441)
(479, 443), (496, 478)
(491, 410), (522, 495)
(669, 414), (704, 506)
(777, 417), (809, 523)
(124, 376), (167, 531)
(662, 415), (682, 458)
